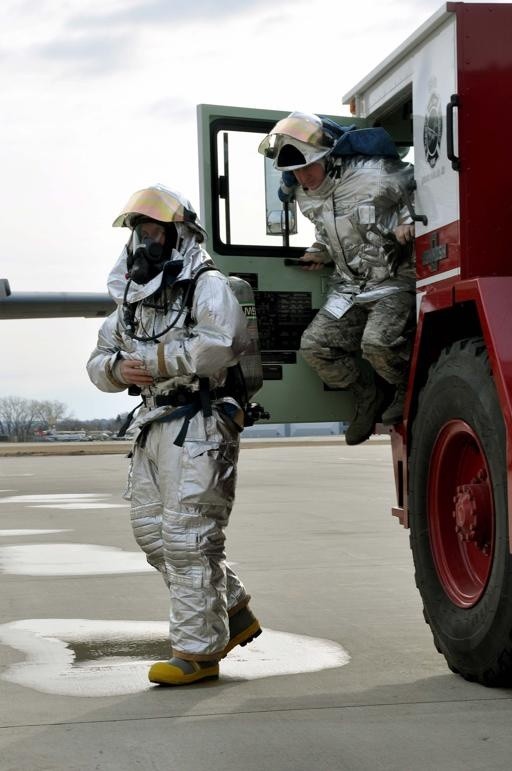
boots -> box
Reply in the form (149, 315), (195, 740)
(343, 369), (388, 446)
(381, 380), (407, 426)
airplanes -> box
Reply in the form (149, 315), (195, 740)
(45, 425), (87, 442)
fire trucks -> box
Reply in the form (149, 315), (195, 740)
(185, 2), (512, 690)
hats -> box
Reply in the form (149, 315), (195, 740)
(257, 111), (339, 174)
(112, 186), (199, 228)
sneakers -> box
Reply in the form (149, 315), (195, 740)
(219, 594), (263, 659)
(147, 647), (220, 687)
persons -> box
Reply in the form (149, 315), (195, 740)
(259, 109), (418, 446)
(83, 186), (268, 686)
